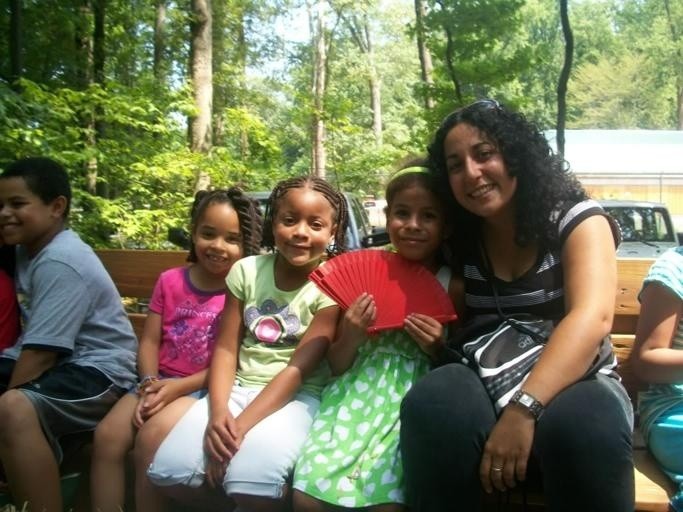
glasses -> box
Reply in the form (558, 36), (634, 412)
(442, 98), (510, 128)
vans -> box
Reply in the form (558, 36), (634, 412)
(244, 192), (388, 253)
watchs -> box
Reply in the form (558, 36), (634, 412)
(509, 389), (545, 421)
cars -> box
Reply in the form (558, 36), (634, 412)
(600, 200), (679, 258)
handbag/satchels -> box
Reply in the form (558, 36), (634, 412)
(461, 311), (620, 421)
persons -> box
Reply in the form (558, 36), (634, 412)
(88, 187), (265, 510)
(0, 156), (138, 511)
(291, 155), (464, 511)
(633, 246), (682, 511)
(144, 177), (349, 511)
(425, 98), (636, 509)
(0, 242), (24, 395)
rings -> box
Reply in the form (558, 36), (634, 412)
(490, 466), (503, 472)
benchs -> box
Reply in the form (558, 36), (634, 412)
(93, 246), (678, 510)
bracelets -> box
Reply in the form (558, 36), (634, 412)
(134, 375), (158, 394)
